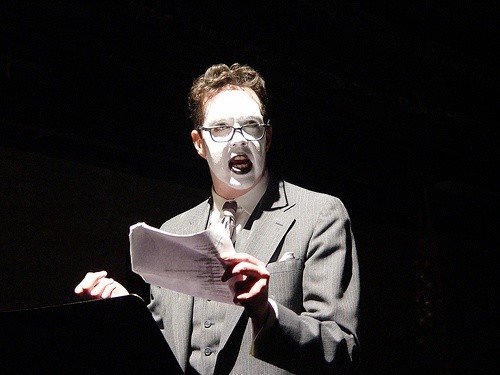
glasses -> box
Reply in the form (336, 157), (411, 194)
(196, 122), (268, 143)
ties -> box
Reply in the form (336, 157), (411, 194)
(218, 200), (238, 249)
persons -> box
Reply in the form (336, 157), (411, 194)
(74, 63), (361, 375)
